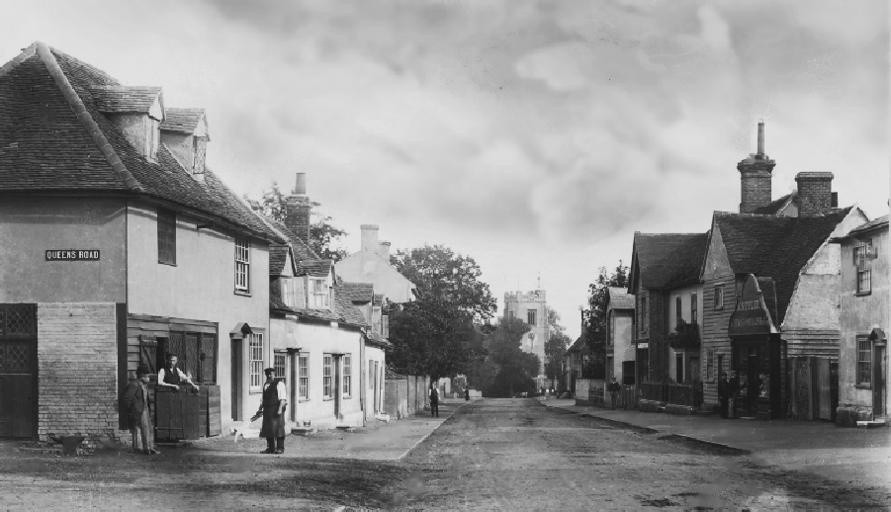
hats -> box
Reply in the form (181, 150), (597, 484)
(136, 364), (150, 374)
(264, 368), (275, 372)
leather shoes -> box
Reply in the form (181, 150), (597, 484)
(274, 448), (284, 454)
(145, 448), (160, 454)
(260, 447), (275, 454)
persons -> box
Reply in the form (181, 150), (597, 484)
(464, 386), (471, 400)
(718, 372), (734, 418)
(158, 353), (200, 391)
(610, 375), (620, 407)
(124, 364), (160, 455)
(255, 367), (289, 455)
(427, 381), (441, 416)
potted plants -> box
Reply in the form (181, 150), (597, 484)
(605, 381), (621, 410)
